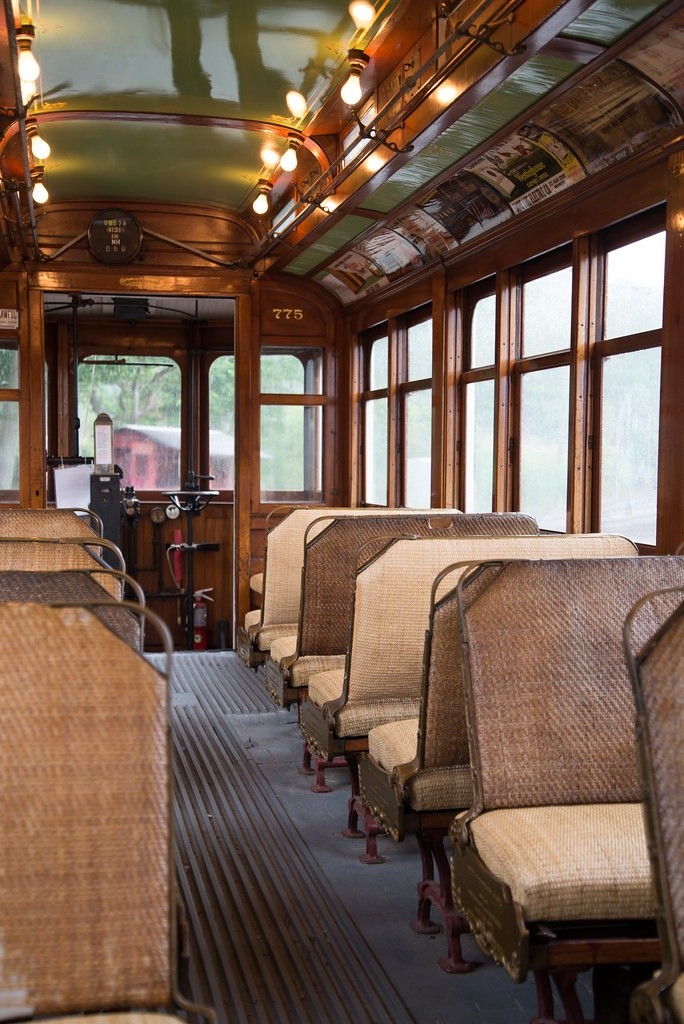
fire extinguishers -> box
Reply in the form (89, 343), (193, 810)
(194, 587), (214, 650)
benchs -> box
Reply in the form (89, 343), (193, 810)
(238, 498), (684, 1024)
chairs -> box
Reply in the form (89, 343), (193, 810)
(0, 508), (220, 1024)
